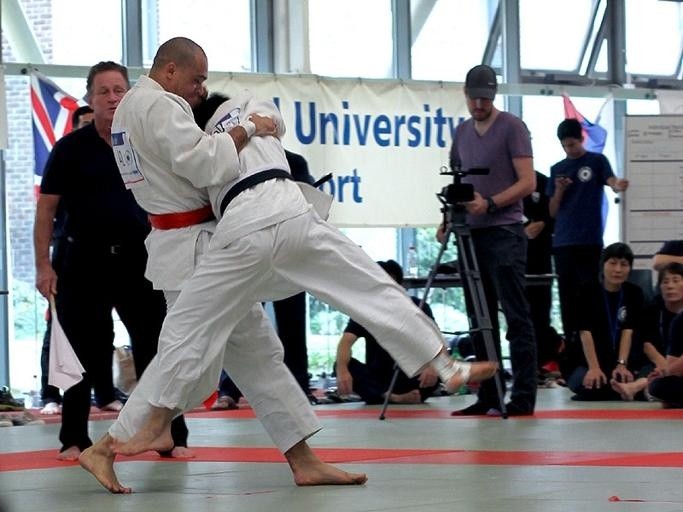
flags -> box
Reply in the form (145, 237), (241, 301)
(29, 65), (91, 212)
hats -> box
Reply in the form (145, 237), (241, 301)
(466, 64), (496, 99)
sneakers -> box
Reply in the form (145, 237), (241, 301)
(0, 386), (45, 426)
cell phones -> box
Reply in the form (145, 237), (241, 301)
(556, 174), (568, 180)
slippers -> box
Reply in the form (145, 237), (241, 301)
(210, 397), (239, 410)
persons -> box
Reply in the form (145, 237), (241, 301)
(436, 64), (683, 416)
(112, 88), (501, 462)
(39, 291), (125, 419)
(68, 105), (93, 128)
(35, 64), (191, 459)
(207, 147), (330, 409)
(331, 258), (445, 404)
(79, 37), (370, 492)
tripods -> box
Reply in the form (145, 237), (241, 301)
(379, 225), (508, 420)
